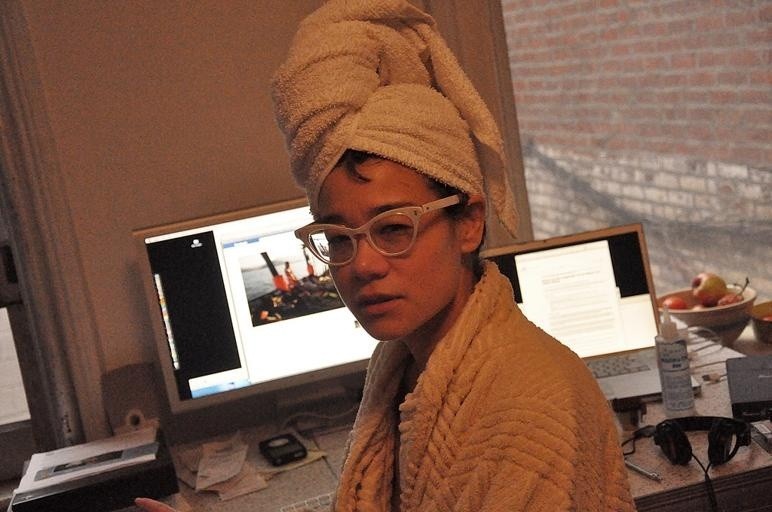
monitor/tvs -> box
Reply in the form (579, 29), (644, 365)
(131, 194), (382, 432)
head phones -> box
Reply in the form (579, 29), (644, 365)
(654, 414), (753, 464)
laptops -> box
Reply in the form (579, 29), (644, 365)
(478, 221), (702, 406)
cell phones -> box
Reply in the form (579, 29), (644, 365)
(259, 434), (308, 466)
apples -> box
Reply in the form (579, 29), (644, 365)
(663, 272), (749, 309)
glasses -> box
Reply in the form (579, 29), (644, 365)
(294, 194), (461, 267)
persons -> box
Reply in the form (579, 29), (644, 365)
(302, 242), (316, 277)
(132, 148), (641, 512)
(253, 269), (341, 323)
(282, 261), (299, 288)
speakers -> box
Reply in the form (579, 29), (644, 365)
(101, 360), (173, 436)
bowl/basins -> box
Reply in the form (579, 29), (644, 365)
(655, 284), (758, 349)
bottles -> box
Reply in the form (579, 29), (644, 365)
(654, 303), (696, 419)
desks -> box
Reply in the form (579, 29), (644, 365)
(113, 312), (770, 512)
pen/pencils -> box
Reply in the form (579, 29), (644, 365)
(624, 460), (662, 481)
